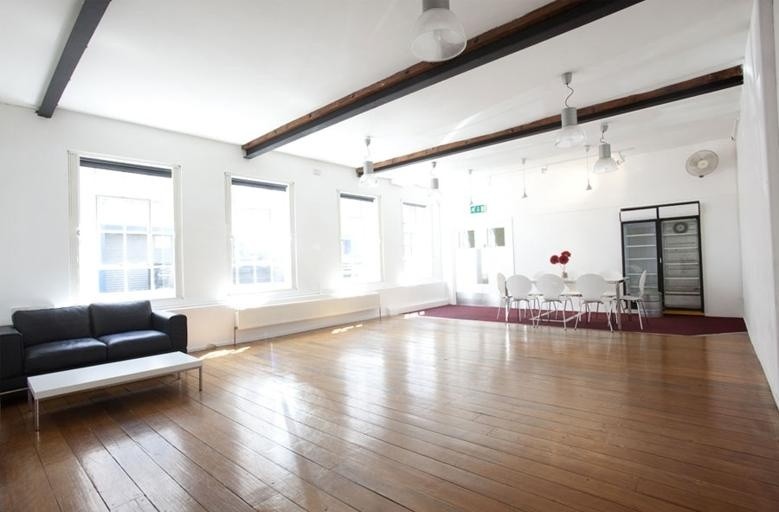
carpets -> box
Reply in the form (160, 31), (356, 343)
(418, 304), (748, 335)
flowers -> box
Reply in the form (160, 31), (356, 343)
(551, 251), (571, 265)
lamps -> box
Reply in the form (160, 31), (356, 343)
(561, 73), (577, 132)
(598, 125), (612, 161)
(358, 136), (378, 187)
(407, 0), (468, 64)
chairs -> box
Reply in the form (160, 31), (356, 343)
(495, 271), (648, 332)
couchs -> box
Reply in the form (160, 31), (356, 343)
(0, 300), (187, 407)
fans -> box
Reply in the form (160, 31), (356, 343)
(686, 148), (719, 178)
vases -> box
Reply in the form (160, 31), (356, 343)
(561, 266), (568, 279)
